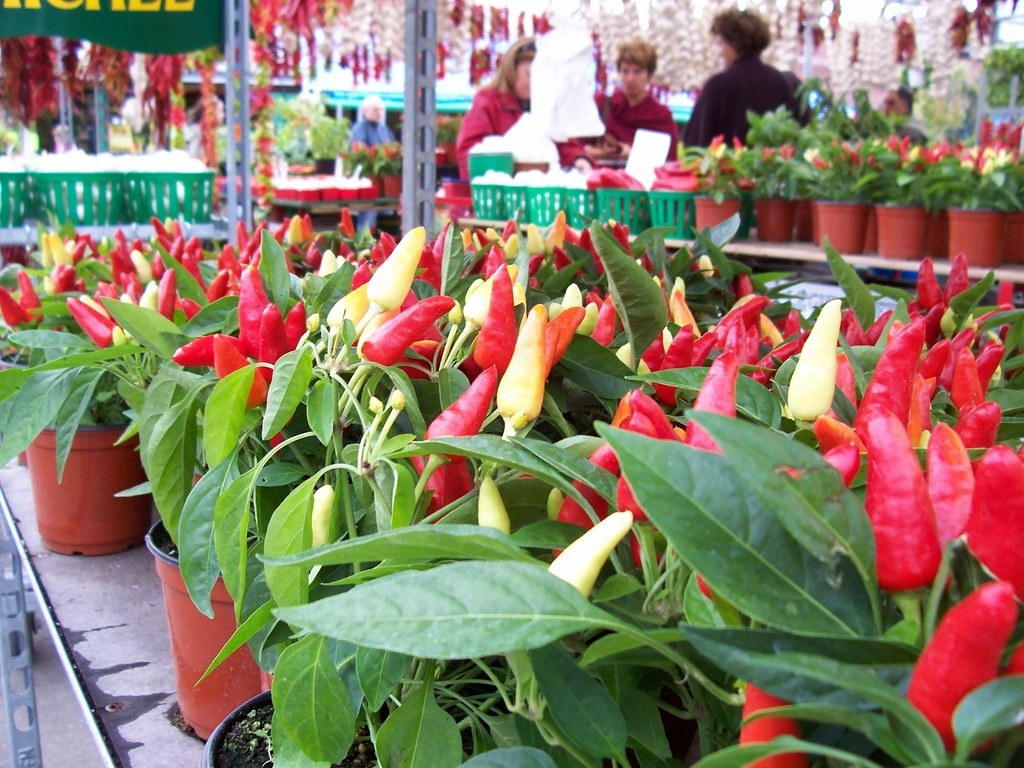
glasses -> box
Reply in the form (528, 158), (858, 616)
(518, 42), (537, 52)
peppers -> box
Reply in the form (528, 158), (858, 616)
(0, 208), (1024, 768)
(774, 0), (997, 66)
(0, 0), (608, 227)
(585, 120), (1024, 190)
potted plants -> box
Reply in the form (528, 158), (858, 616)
(0, 115), (1024, 766)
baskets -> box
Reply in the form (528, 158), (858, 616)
(0, 166), (216, 227)
(469, 180), (700, 242)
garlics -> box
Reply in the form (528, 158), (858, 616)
(338, 0), (961, 94)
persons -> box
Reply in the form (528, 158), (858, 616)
(883, 89), (927, 145)
(349, 95), (397, 231)
(186, 94), (224, 157)
(578, 40), (679, 158)
(456, 37), (595, 181)
(683, 6), (809, 149)
